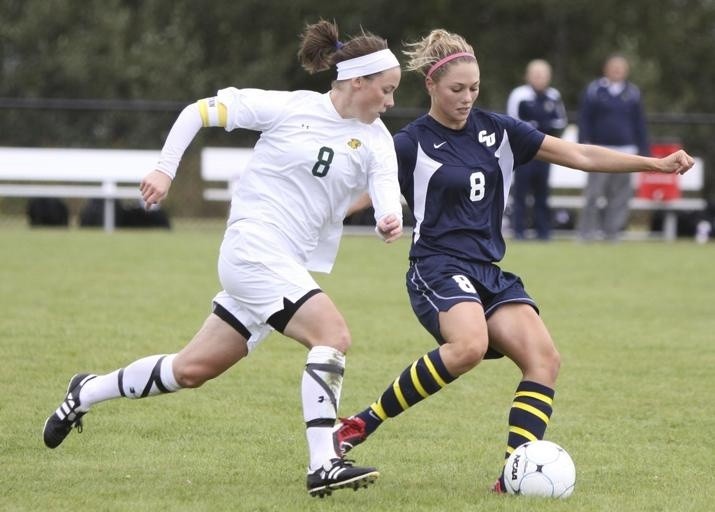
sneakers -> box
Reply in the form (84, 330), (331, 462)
(43, 373), (97, 448)
(306, 458), (379, 498)
(333, 417), (368, 458)
(492, 479), (506, 493)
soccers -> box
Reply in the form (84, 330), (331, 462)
(504, 441), (576, 499)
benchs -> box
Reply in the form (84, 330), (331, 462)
(0, 146), (180, 235)
(200, 148), (708, 242)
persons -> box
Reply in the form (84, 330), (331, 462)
(576, 54), (653, 245)
(506, 57), (568, 243)
(40, 13), (404, 498)
(333, 26), (696, 491)
